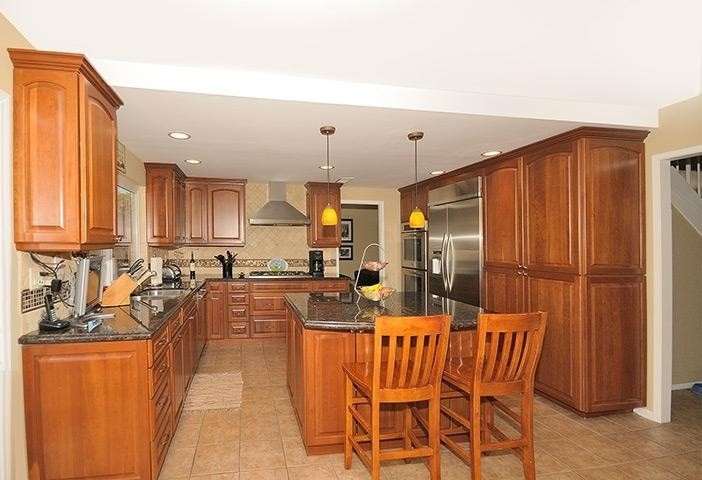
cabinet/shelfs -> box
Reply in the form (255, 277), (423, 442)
(142, 279), (207, 367)
(145, 163), (185, 248)
(94, 298), (195, 420)
(251, 279), (351, 339)
(483, 126), (650, 275)
(207, 279), (228, 341)
(228, 279), (251, 339)
(21, 317), (172, 479)
(484, 273), (648, 420)
(8, 48), (123, 253)
(185, 176), (247, 248)
(399, 185), (429, 222)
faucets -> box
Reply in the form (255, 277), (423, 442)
(139, 271), (157, 292)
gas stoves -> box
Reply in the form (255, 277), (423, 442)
(249, 271), (312, 278)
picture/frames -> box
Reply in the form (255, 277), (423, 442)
(341, 219), (353, 243)
(339, 245), (352, 260)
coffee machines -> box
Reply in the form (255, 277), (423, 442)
(309, 250), (324, 280)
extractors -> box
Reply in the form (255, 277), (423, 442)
(249, 182), (310, 226)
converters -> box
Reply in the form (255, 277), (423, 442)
(51, 279), (61, 292)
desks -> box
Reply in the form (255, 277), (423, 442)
(283, 291), (493, 457)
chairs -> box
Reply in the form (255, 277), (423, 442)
(342, 314), (453, 480)
(441, 312), (548, 480)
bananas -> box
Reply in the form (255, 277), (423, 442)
(358, 281), (384, 296)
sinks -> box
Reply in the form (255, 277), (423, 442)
(130, 287), (186, 298)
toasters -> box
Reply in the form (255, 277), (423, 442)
(162, 264), (181, 280)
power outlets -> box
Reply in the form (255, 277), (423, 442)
(304, 182), (344, 248)
(29, 267), (47, 289)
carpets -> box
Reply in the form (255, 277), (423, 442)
(182, 372), (244, 412)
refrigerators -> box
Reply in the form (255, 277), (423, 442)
(427, 198), (482, 307)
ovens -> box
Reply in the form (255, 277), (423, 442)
(400, 232), (427, 270)
(400, 267), (427, 292)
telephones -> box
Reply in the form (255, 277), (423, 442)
(39, 294), (70, 330)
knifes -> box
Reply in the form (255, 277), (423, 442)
(128, 264), (143, 276)
(120, 271), (157, 304)
(127, 258), (144, 273)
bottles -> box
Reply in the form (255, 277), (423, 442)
(190, 251), (195, 279)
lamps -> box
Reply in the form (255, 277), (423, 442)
(320, 126), (338, 225)
(408, 132), (424, 228)
